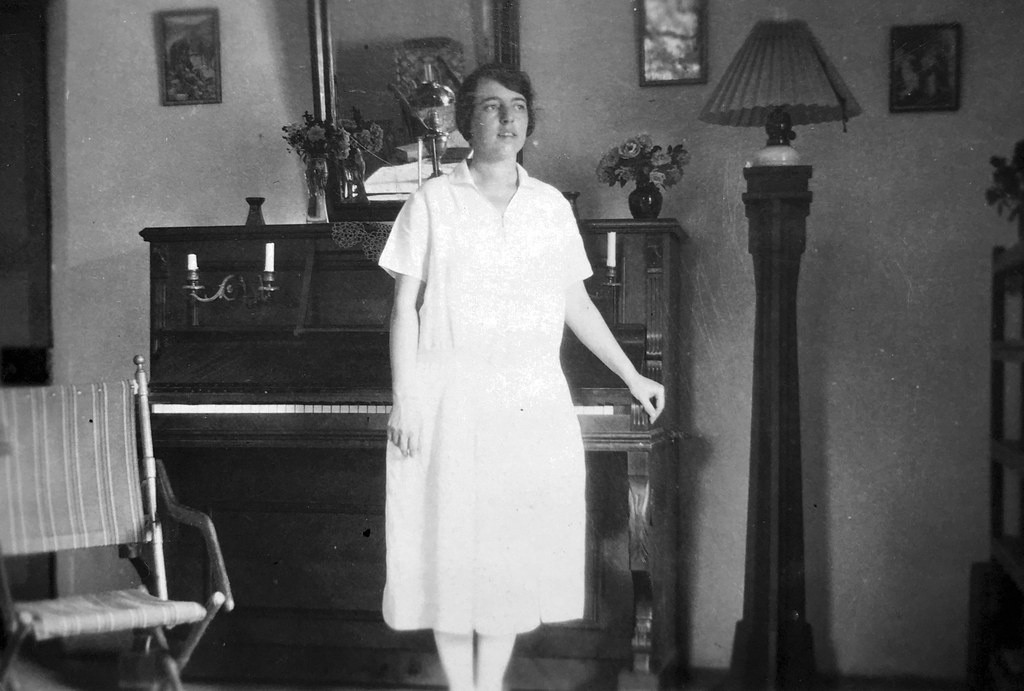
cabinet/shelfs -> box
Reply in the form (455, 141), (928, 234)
(964, 240), (1024, 691)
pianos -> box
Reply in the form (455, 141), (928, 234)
(128, 215), (695, 691)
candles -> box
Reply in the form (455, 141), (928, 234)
(187, 254), (197, 269)
(606, 232), (617, 268)
(264, 242), (275, 272)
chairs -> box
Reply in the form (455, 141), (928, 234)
(0, 355), (235, 691)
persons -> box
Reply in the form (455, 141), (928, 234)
(377, 63), (667, 690)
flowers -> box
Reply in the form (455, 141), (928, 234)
(595, 132), (692, 191)
(337, 118), (384, 156)
(281, 113), (350, 165)
(987, 140), (1024, 223)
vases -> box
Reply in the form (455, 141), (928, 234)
(304, 158), (329, 224)
(343, 148), (371, 205)
(628, 182), (663, 221)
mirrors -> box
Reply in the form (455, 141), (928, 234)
(307, 0), (524, 222)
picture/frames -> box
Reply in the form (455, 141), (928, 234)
(153, 6), (222, 106)
(633, 0), (708, 87)
(888, 21), (963, 113)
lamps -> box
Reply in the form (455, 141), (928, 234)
(694, 16), (863, 166)
